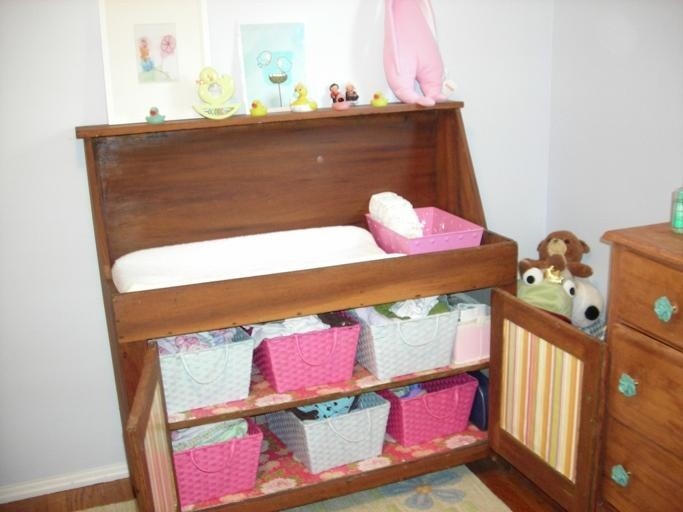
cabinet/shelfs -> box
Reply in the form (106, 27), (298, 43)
(598, 218), (683, 511)
(76, 100), (607, 512)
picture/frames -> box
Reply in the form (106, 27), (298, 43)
(234, 18), (312, 118)
(96, 0), (213, 125)
